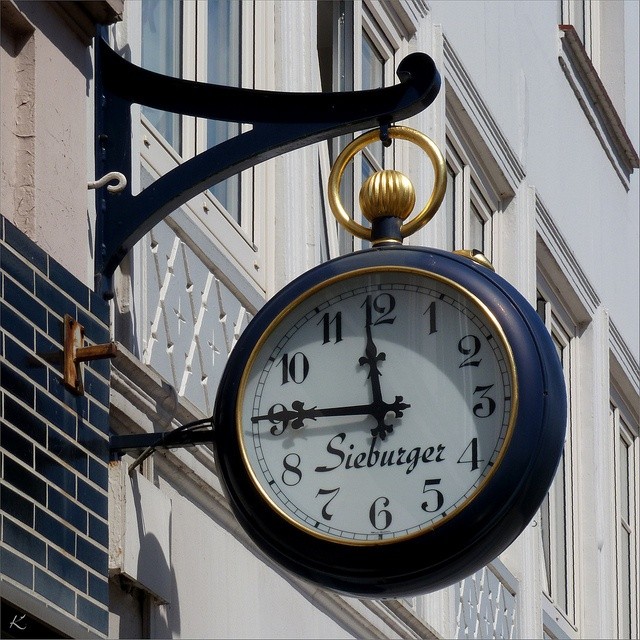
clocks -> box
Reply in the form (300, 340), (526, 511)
(211, 129), (567, 600)
